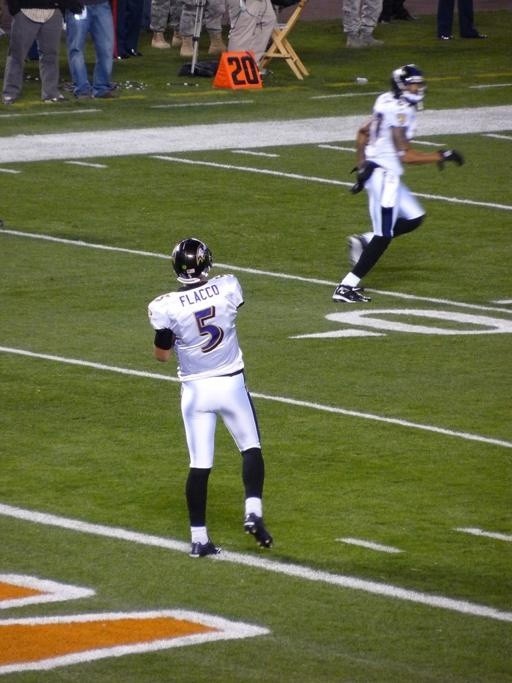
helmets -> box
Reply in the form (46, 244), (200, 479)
(172, 238), (212, 284)
(391, 62), (426, 104)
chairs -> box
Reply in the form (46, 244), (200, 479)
(257, 0), (310, 81)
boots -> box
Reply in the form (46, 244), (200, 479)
(152, 31), (227, 55)
(360, 31), (383, 48)
(347, 34), (368, 49)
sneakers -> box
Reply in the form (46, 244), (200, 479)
(244, 513), (272, 547)
(3, 91), (118, 102)
(331, 284), (371, 303)
(189, 535), (222, 558)
(349, 234), (368, 267)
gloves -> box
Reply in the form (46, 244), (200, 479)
(440, 148), (462, 165)
(350, 167), (362, 193)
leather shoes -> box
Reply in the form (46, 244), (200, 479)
(130, 48), (139, 56)
(440, 32), (454, 39)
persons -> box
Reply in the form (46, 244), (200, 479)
(1, 2), (488, 103)
(147, 237), (273, 558)
(330, 63), (464, 303)
